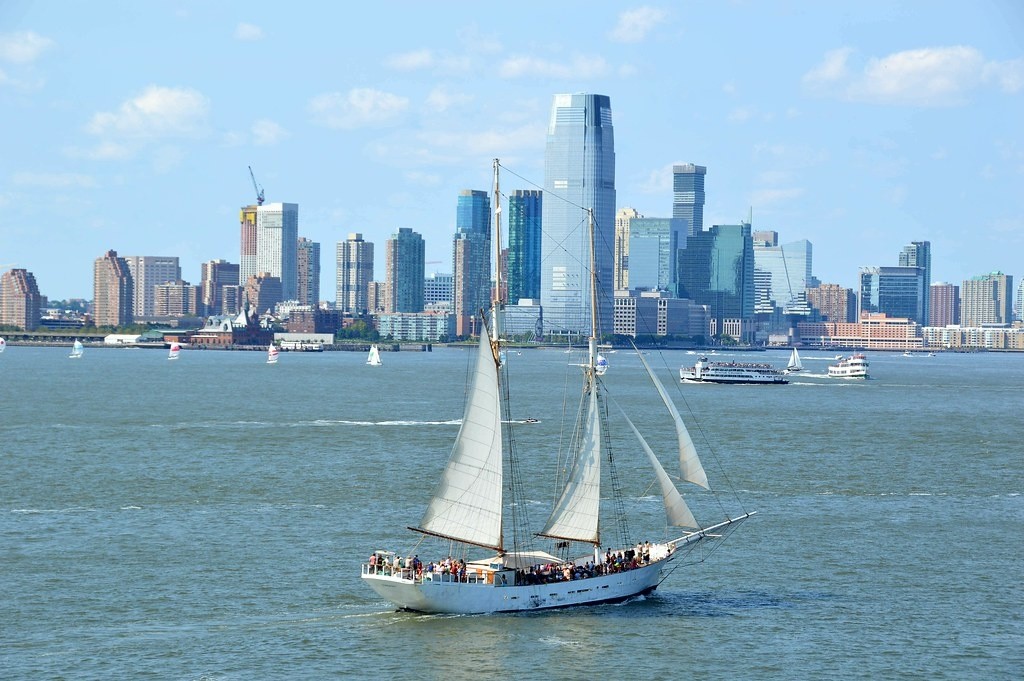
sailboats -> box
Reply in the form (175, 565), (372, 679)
(167, 340), (180, 360)
(787, 347), (804, 371)
(361, 158), (761, 618)
(68, 338), (84, 358)
(366, 344), (382, 365)
(266, 343), (278, 364)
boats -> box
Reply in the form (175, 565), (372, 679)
(904, 349), (912, 356)
(679, 356), (790, 385)
(928, 352), (935, 357)
(828, 352), (871, 380)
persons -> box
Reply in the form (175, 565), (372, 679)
(368, 540), (652, 586)
